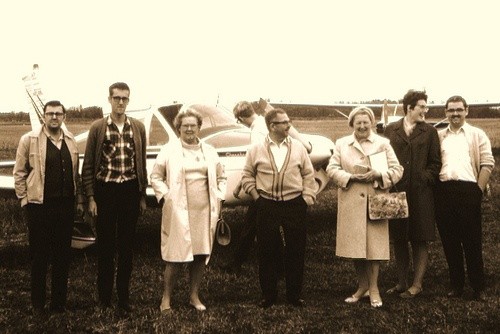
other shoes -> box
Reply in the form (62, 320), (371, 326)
(32, 306), (46, 314)
(117, 303), (136, 311)
(386, 285), (422, 298)
(371, 300), (383, 307)
(260, 298), (278, 308)
(448, 289), (464, 297)
(49, 303), (66, 312)
(289, 296), (306, 306)
(344, 290), (369, 302)
(160, 306), (174, 316)
(104, 303), (115, 311)
(476, 290), (487, 301)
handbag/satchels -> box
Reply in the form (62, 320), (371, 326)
(216, 202), (231, 246)
(71, 211), (96, 248)
(366, 171), (409, 220)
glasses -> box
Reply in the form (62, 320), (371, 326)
(237, 119), (242, 124)
(273, 119), (292, 124)
(45, 112), (64, 116)
(416, 105), (429, 111)
(446, 108), (465, 112)
(111, 97), (129, 102)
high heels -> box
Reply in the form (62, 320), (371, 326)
(190, 300), (206, 311)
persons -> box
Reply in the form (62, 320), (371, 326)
(233, 100), (270, 199)
(326, 107), (404, 308)
(150, 109), (228, 313)
(82, 82), (147, 319)
(438, 96), (496, 301)
(242, 108), (318, 308)
(12, 100), (79, 318)
(381, 89), (442, 299)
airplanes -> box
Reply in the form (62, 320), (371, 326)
(0, 60), (332, 268)
(265, 95), (499, 137)
